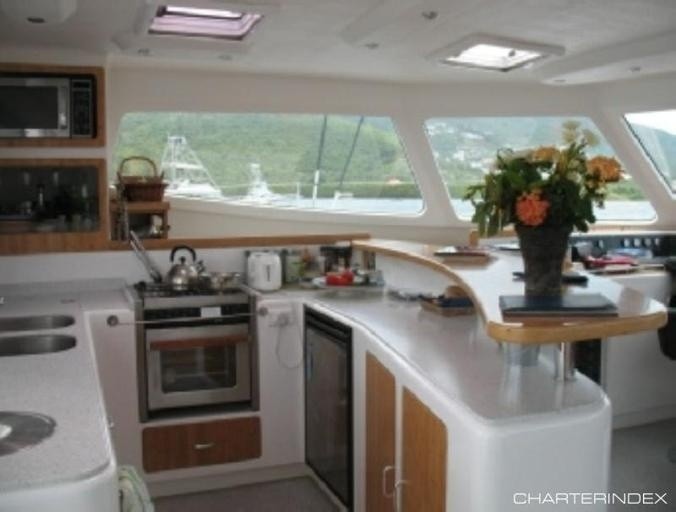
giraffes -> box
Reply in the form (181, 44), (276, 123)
(139, 281), (251, 321)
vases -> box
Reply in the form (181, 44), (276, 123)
(463, 117), (628, 239)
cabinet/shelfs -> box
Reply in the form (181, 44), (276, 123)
(18, 178), (95, 229)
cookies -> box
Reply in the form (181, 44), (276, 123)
(1, 64), (99, 142)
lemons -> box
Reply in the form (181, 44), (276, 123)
(166, 242), (207, 292)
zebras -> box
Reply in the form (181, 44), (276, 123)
(144, 324), (259, 415)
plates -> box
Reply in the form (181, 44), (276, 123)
(0, 158), (107, 257)
(140, 411), (263, 479)
(356, 330), (477, 512)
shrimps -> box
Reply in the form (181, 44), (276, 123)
(246, 250), (282, 292)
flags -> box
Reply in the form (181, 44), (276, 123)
(512, 220), (576, 293)
